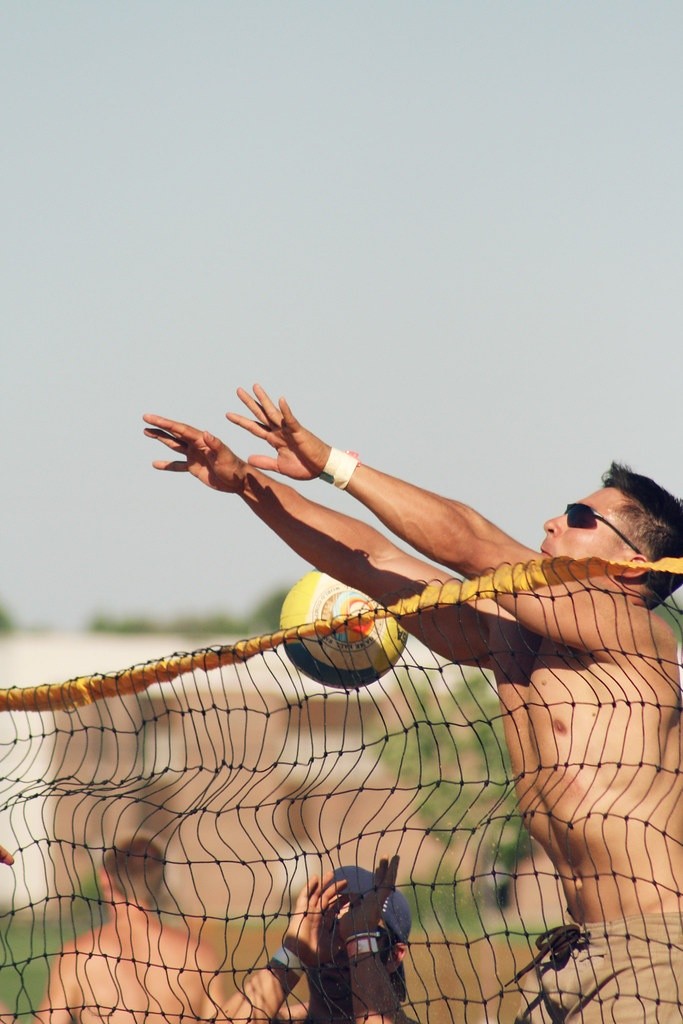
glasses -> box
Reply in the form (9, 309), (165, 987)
(565, 503), (642, 556)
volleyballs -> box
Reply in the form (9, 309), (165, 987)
(278, 568), (409, 691)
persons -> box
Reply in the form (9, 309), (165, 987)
(209, 855), (425, 1024)
(0, 846), (18, 1024)
(140, 384), (683, 1024)
(39, 837), (225, 1024)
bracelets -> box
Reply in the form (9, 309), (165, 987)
(344, 931), (380, 945)
(346, 937), (379, 958)
(320, 446), (361, 491)
(274, 944), (308, 978)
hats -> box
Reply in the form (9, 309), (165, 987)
(321, 865), (412, 1002)
